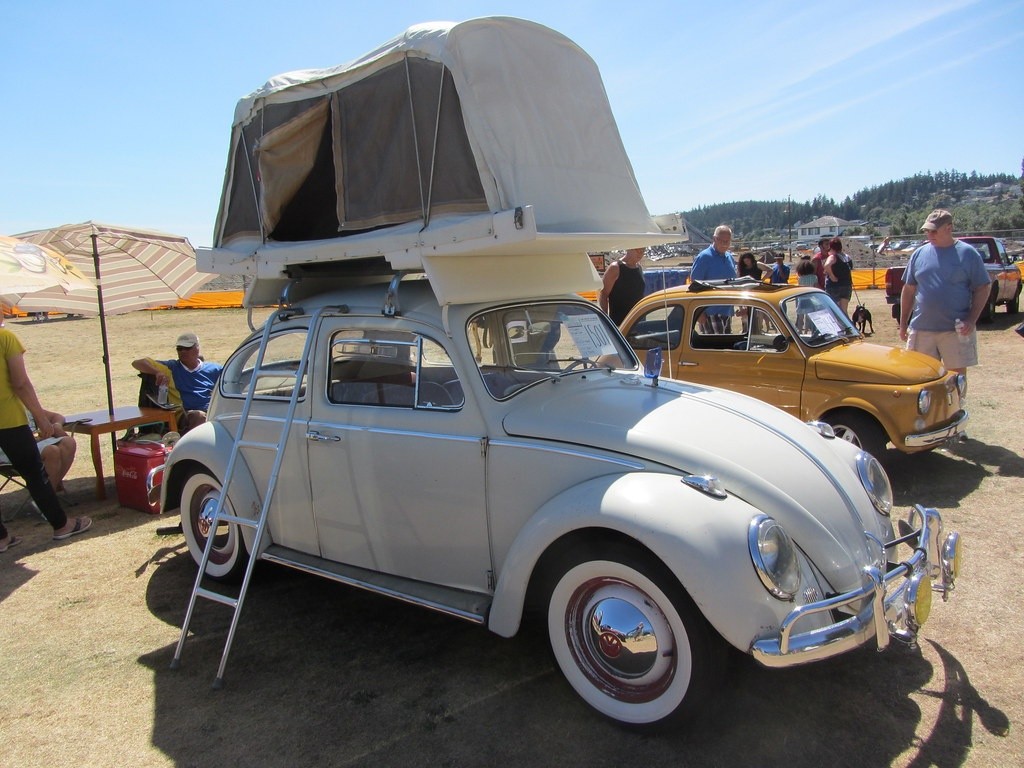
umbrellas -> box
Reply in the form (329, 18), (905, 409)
(0, 235), (98, 293)
(0, 219), (220, 453)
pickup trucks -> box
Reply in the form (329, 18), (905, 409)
(884, 236), (1023, 323)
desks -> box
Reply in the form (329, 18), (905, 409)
(62, 406), (179, 501)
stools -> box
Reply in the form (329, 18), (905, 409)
(0, 465), (80, 523)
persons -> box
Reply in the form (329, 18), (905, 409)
(599, 247), (646, 326)
(0, 303), (92, 551)
(0, 408), (77, 490)
(796, 237), (853, 334)
(132, 333), (223, 429)
(692, 226), (738, 334)
(738, 251), (790, 334)
(901, 209), (992, 439)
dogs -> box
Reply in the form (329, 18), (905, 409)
(851, 302), (874, 335)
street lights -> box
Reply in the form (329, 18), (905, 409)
(783, 195), (793, 262)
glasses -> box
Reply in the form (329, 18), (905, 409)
(175, 346), (192, 352)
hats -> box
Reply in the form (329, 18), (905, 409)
(918, 209), (954, 234)
(774, 254), (785, 260)
(176, 332), (199, 347)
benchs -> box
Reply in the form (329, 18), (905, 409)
(242, 380), (342, 397)
(444, 373), (508, 407)
(629, 329), (680, 349)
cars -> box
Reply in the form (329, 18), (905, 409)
(864, 238), (923, 260)
(750, 239), (811, 254)
(145, 276), (964, 736)
(586, 276), (971, 470)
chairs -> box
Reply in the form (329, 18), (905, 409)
(419, 381), (454, 407)
(734, 341), (750, 350)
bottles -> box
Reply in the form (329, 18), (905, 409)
(157, 377), (168, 404)
(955, 319), (969, 343)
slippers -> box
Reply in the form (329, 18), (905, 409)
(1, 536), (23, 554)
(52, 516), (95, 540)
